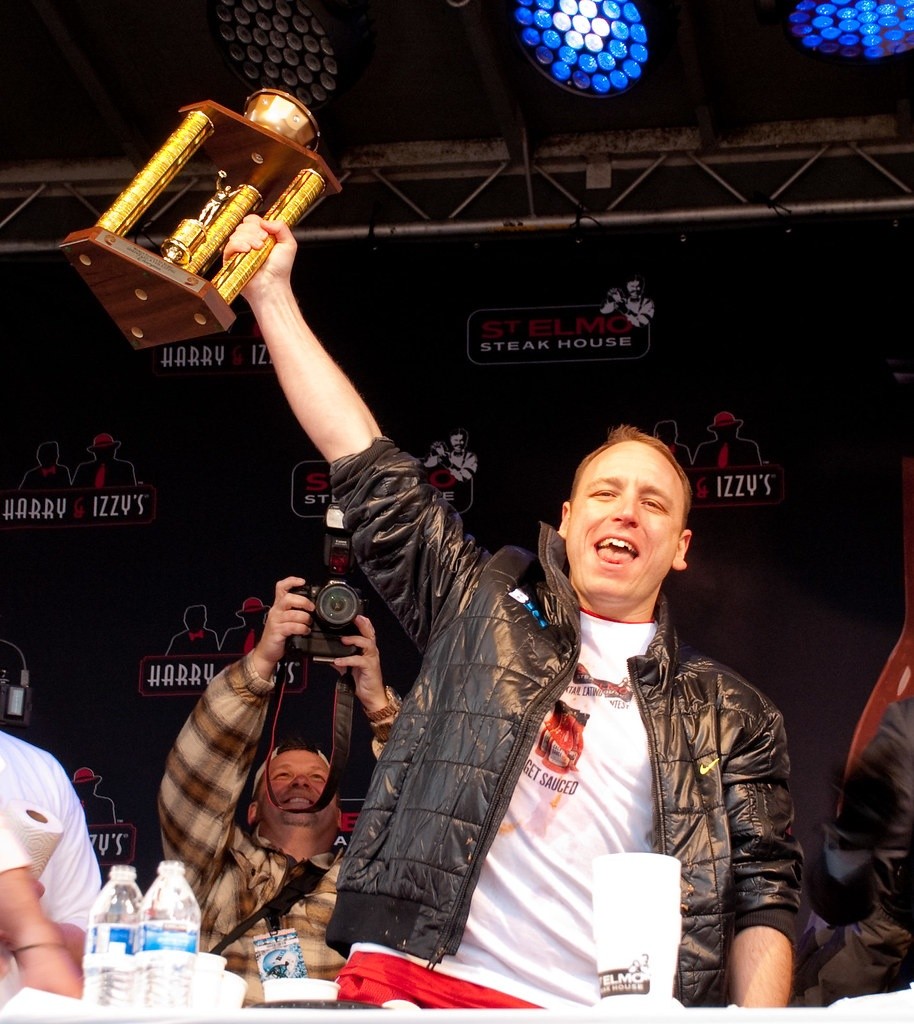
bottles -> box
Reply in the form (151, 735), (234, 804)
(81, 864), (143, 1010)
(128, 860), (202, 1012)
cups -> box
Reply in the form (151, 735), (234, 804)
(186, 951), (249, 1010)
(262, 978), (342, 1003)
(590, 851), (684, 1009)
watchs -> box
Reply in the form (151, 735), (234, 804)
(363, 686), (403, 720)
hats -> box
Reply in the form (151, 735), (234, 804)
(252, 742), (330, 798)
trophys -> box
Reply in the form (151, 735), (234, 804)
(61, 87), (341, 350)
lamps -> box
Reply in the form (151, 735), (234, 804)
(505, 0), (683, 99)
(753, 0), (914, 68)
(204, 0), (378, 113)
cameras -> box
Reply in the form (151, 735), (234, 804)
(287, 503), (364, 657)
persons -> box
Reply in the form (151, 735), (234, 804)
(810, 696), (914, 1003)
(157, 576), (404, 1007)
(0, 730), (104, 999)
(222, 212), (802, 1008)
